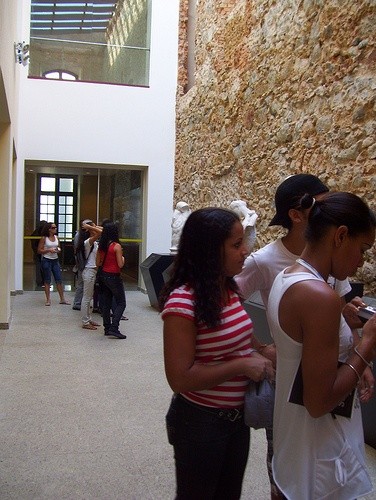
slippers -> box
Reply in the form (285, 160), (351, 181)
(46, 301), (51, 306)
(60, 301), (72, 305)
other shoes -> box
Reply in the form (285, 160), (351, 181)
(93, 308), (101, 313)
(110, 314), (113, 317)
(82, 324), (98, 330)
(72, 306), (81, 310)
(108, 330), (126, 339)
(121, 316), (127, 320)
(90, 321), (100, 326)
(104, 332), (109, 336)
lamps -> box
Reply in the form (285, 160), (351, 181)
(14, 40), (31, 67)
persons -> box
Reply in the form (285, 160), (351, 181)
(270, 190), (376, 500)
(230, 174), (352, 500)
(161, 208), (274, 499)
(31, 218), (129, 339)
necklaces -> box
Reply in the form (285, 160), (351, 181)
(296, 257), (325, 282)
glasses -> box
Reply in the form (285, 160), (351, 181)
(50, 226), (57, 229)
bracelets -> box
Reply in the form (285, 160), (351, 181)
(355, 345), (373, 369)
(343, 363), (361, 384)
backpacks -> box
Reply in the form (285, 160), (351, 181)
(76, 238), (94, 270)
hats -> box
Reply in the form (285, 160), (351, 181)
(268, 175), (329, 227)
